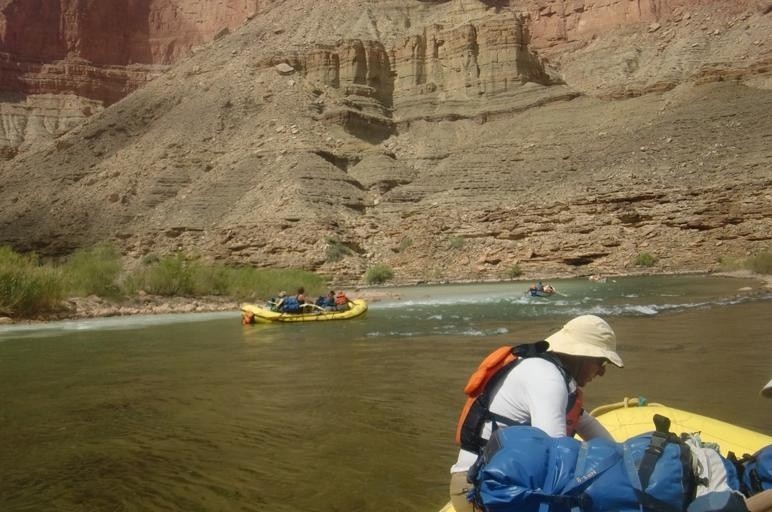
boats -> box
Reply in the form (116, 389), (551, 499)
(439, 395), (772, 510)
(525, 286), (554, 297)
(241, 298), (371, 322)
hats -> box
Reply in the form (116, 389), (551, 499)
(544, 315), (624, 368)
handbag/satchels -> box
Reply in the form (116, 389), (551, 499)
(467, 425), (696, 511)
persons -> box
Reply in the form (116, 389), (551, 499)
(529, 281), (553, 293)
(272, 287), (359, 313)
(449, 314), (625, 512)
(744, 379), (772, 512)
